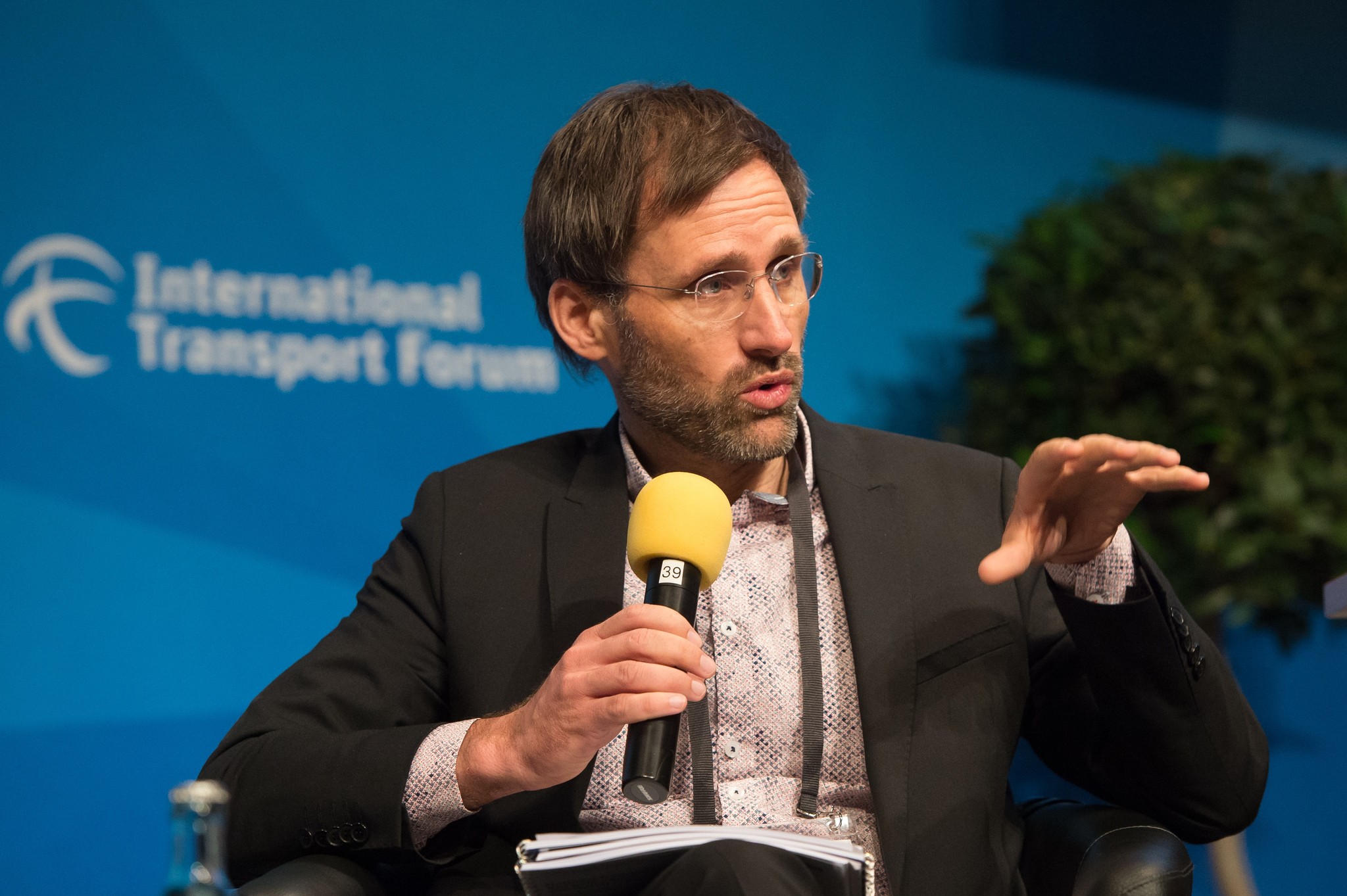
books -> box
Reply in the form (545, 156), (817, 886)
(514, 824), (875, 896)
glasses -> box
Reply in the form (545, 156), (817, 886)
(599, 251), (827, 325)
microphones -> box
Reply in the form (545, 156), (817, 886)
(620, 472), (732, 805)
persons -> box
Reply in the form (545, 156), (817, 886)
(192, 83), (1271, 896)
